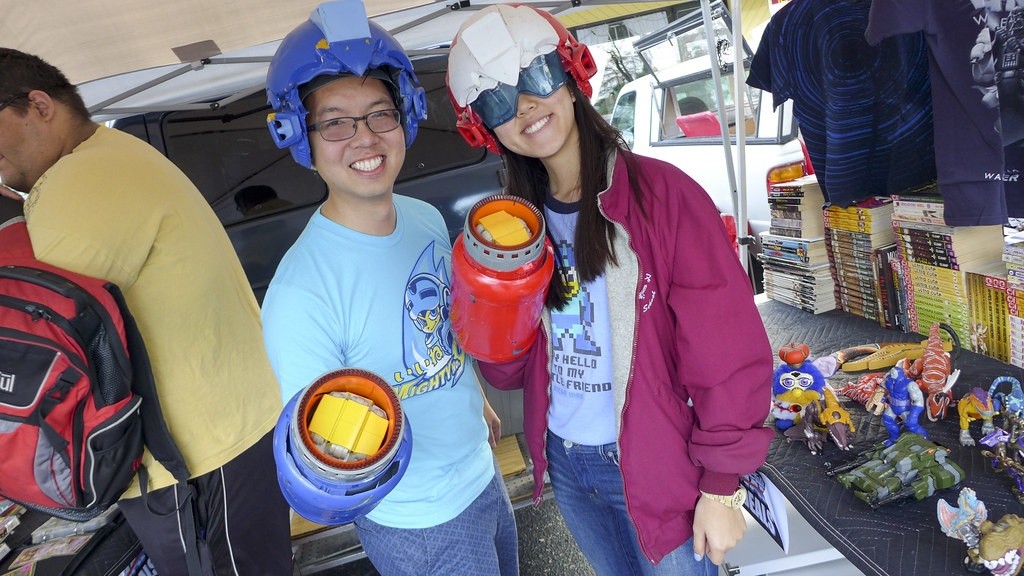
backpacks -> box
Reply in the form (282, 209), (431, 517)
(0, 199), (196, 524)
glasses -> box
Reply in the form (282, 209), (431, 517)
(307, 108), (401, 141)
(470, 50), (570, 130)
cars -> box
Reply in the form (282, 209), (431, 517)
(109, 48), (506, 305)
(606, 1), (816, 298)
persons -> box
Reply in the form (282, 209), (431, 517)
(0, 48), (294, 576)
(259, 1), (520, 576)
(445, 4), (776, 576)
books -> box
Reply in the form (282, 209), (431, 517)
(757, 172), (1024, 370)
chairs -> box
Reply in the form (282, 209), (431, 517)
(675, 111), (721, 137)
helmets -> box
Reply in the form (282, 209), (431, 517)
(445, 3), (597, 153)
(267, 0), (430, 170)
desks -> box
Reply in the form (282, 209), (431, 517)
(754, 299), (1024, 576)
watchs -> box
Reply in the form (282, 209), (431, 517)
(700, 483), (747, 510)
